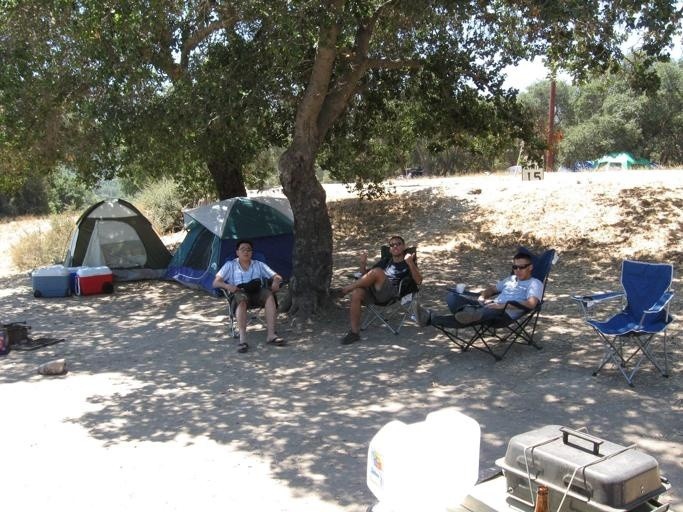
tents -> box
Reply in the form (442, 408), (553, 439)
(58, 197), (174, 283)
(575, 150), (660, 172)
(165, 195), (297, 297)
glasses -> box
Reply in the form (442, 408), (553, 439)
(512, 263), (532, 269)
(239, 248), (252, 252)
(389, 242), (402, 247)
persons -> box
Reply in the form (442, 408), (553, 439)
(210, 241), (284, 354)
(557, 161), (568, 172)
(410, 252), (545, 330)
(328, 236), (423, 344)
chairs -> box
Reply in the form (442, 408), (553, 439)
(346, 246), (428, 338)
(570, 259), (679, 387)
(214, 253), (277, 339)
(429, 246), (561, 362)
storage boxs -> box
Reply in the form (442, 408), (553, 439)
(30, 262), (114, 298)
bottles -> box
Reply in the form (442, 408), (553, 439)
(532, 485), (549, 512)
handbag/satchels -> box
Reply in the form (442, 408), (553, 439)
(244, 278), (261, 292)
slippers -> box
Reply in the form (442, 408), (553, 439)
(268, 337), (285, 346)
(238, 343), (248, 352)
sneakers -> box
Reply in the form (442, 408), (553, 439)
(412, 300), (428, 327)
(343, 332), (360, 344)
(455, 309), (482, 325)
(329, 288), (345, 298)
